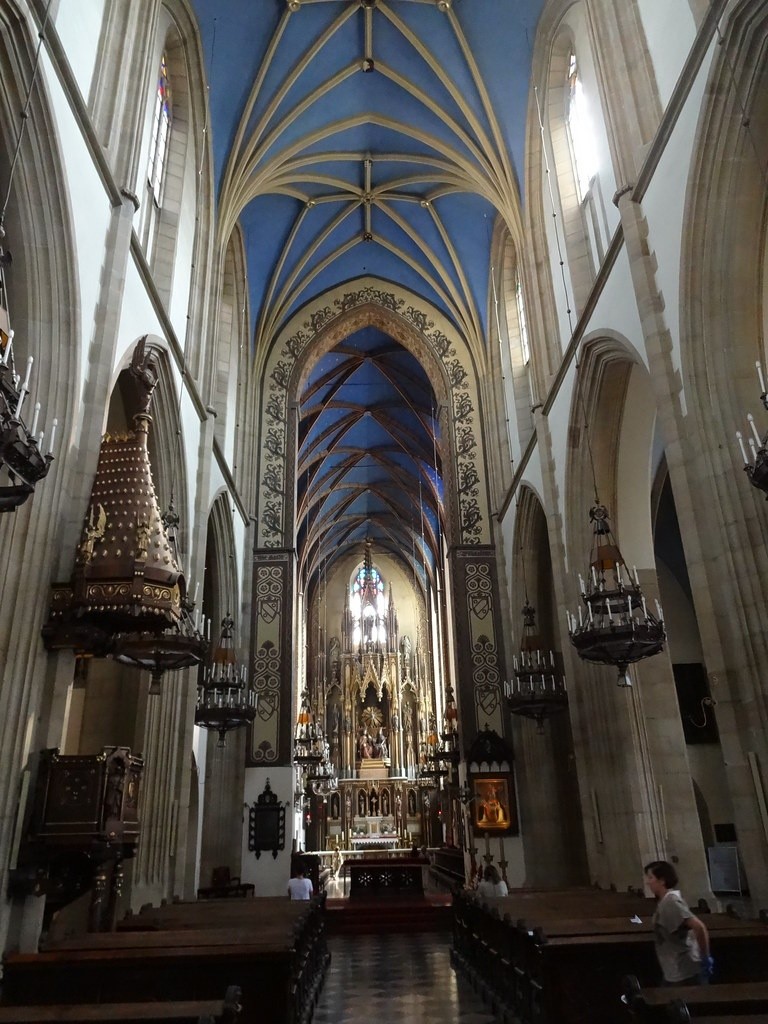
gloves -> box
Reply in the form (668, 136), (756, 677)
(700, 951), (713, 975)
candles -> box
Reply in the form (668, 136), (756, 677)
(349, 829), (351, 838)
(398, 827), (400, 836)
(336, 834), (338, 844)
(342, 832), (344, 841)
(404, 830), (406, 839)
(409, 832), (411, 841)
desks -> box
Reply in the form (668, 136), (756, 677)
(299, 847), (442, 882)
(342, 858), (431, 899)
(349, 838), (398, 850)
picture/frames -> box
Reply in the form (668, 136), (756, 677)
(467, 771), (520, 837)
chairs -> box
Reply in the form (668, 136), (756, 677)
(199, 867), (255, 898)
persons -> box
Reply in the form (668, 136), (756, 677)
(358, 727), (387, 758)
(418, 845), (430, 858)
(287, 862), (314, 900)
(410, 846), (419, 857)
(643, 861), (716, 986)
(475, 866), (508, 897)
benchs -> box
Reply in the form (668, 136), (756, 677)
(450, 887), (768, 1024)
(0, 890), (331, 1024)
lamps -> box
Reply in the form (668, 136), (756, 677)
(420, 684), (463, 782)
(483, 588), (572, 734)
(522, 492), (668, 690)
(292, 686), (339, 804)
(0, 214), (59, 511)
(196, 613), (261, 750)
(115, 493), (215, 696)
(735, 360), (768, 501)
